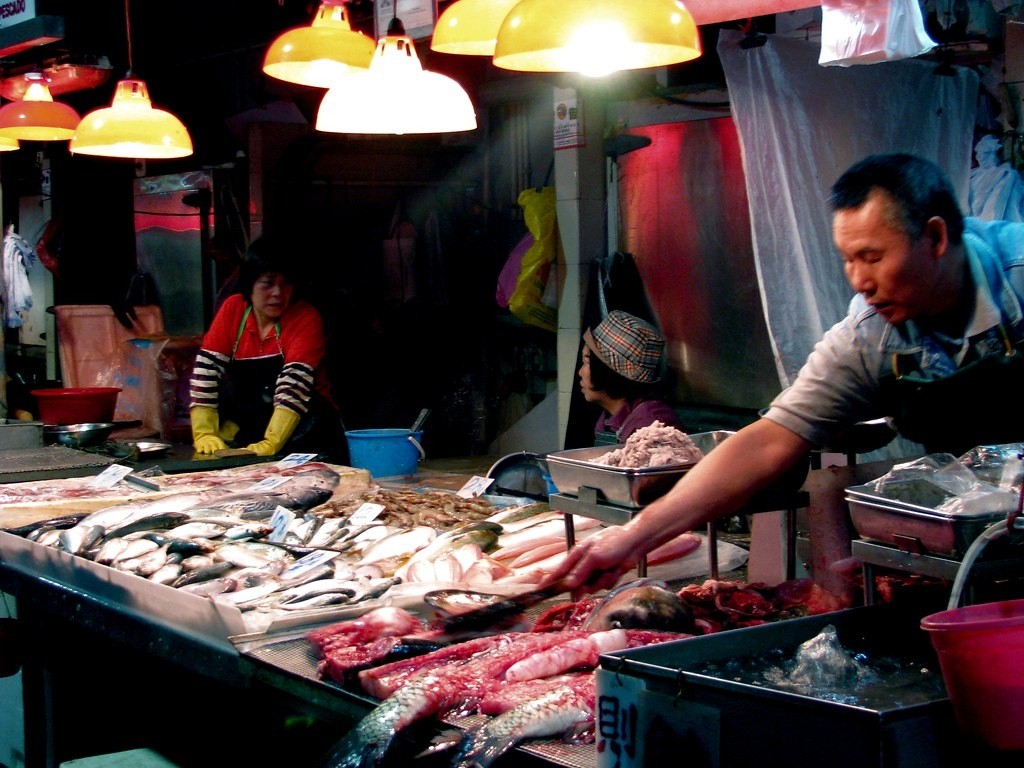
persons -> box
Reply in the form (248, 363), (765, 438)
(189, 236), (345, 466)
(535, 153), (1024, 603)
(578, 310), (687, 447)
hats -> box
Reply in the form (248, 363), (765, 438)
(585, 311), (665, 384)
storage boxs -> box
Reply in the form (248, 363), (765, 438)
(0, 418), (45, 451)
(589, 602), (951, 768)
(529, 427), (737, 510)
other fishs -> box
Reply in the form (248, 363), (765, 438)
(0, 450), (749, 768)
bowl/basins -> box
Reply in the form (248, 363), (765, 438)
(40, 422), (114, 447)
(29, 386), (123, 425)
(483, 450), (549, 502)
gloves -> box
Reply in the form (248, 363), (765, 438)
(239, 408), (300, 458)
(189, 406), (229, 455)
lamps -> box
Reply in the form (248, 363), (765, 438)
(314, 0), (479, 137)
(0, 58), (88, 140)
(0, 137), (21, 152)
(67, 0), (194, 163)
(427, 0), (526, 58)
(258, 0), (377, 89)
(488, 0), (705, 79)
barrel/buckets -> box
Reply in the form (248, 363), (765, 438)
(344, 427), (426, 483)
(920, 515), (1024, 756)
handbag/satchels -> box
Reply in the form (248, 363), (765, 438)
(494, 183), (561, 331)
